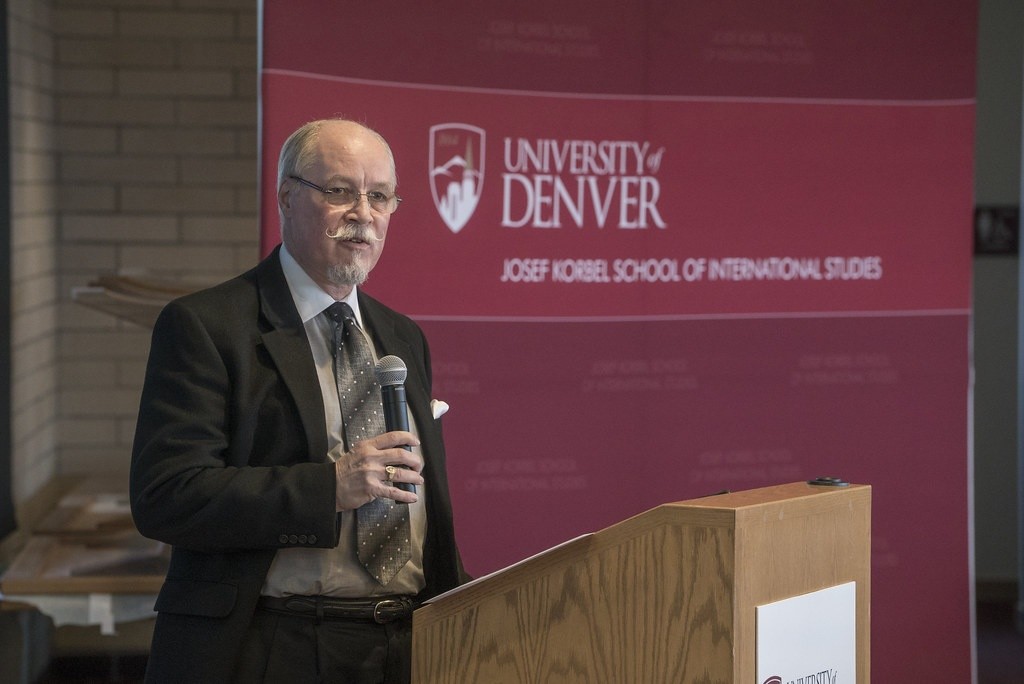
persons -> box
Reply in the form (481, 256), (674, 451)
(129, 118), (459, 684)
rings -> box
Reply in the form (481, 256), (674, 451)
(386, 465), (396, 481)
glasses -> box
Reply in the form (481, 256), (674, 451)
(287, 175), (402, 214)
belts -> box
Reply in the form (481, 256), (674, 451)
(260, 597), (419, 622)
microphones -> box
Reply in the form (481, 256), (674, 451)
(374, 355), (417, 505)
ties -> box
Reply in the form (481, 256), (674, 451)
(323, 303), (411, 585)
